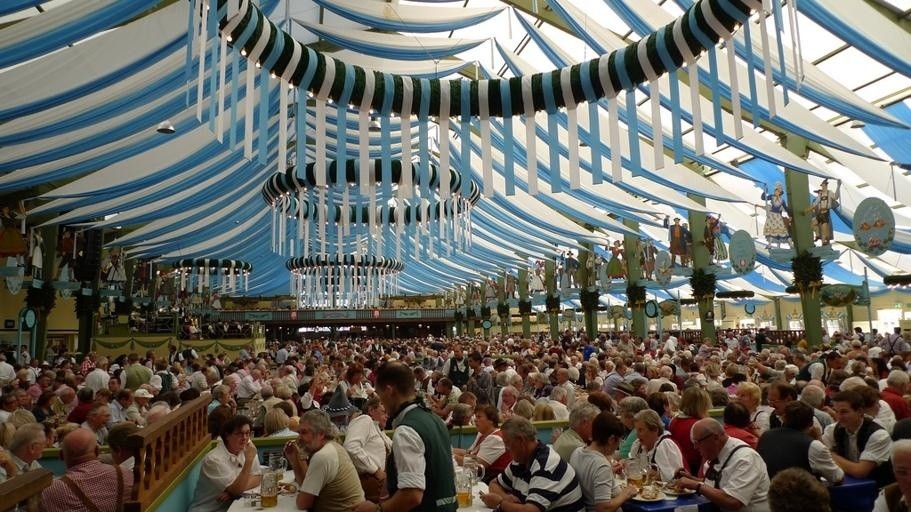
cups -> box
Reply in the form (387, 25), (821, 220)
(462, 453), (486, 487)
(260, 469), (281, 507)
(269, 451), (288, 481)
(455, 473), (474, 509)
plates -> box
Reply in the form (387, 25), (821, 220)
(622, 452), (698, 503)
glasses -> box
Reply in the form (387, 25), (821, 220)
(695, 433), (712, 445)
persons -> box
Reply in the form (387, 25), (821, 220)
(0, 179), (842, 311)
(1, 302), (911, 510)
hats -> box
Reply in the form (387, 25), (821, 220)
(322, 384), (358, 417)
(135, 389), (154, 397)
(327, 342), (335, 348)
(147, 405), (171, 425)
(851, 339), (862, 346)
(867, 347), (882, 359)
(494, 358), (507, 368)
